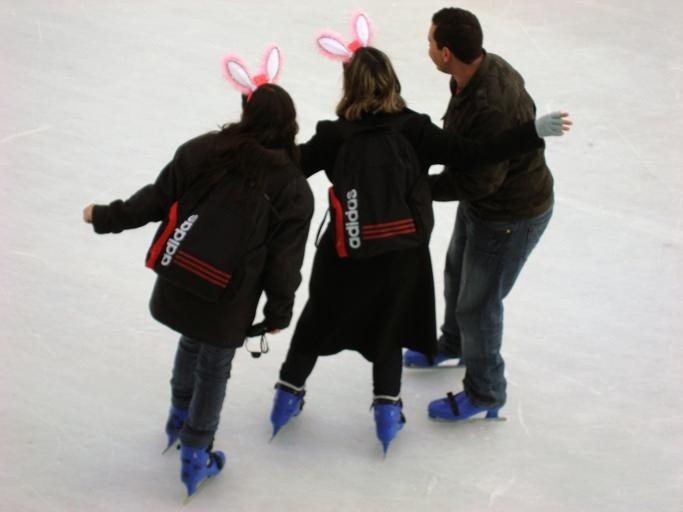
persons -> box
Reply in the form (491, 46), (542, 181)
(403, 6), (555, 423)
(267, 46), (575, 453)
(83, 83), (315, 496)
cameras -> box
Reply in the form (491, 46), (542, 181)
(246, 319), (271, 337)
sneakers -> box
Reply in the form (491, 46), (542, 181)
(429, 391), (504, 416)
(270, 386), (302, 424)
(404, 348), (449, 367)
(165, 410), (183, 437)
(180, 448), (224, 483)
(374, 407), (405, 442)
